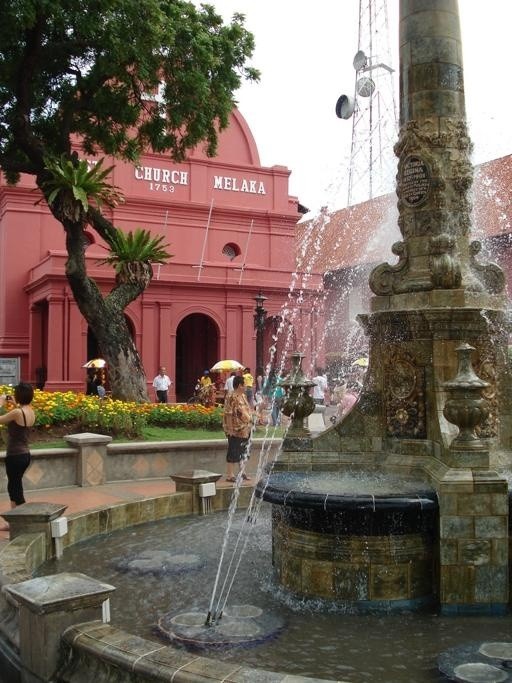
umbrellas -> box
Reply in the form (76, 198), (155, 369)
(81, 359), (106, 368)
(210, 360), (245, 380)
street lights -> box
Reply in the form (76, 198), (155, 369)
(250, 288), (268, 411)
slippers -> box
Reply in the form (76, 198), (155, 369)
(226, 476), (237, 482)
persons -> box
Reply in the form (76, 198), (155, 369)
(0, 383), (35, 509)
(86, 367), (362, 425)
(222, 378), (252, 481)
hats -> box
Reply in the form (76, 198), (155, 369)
(204, 370), (209, 376)
(243, 367), (250, 371)
